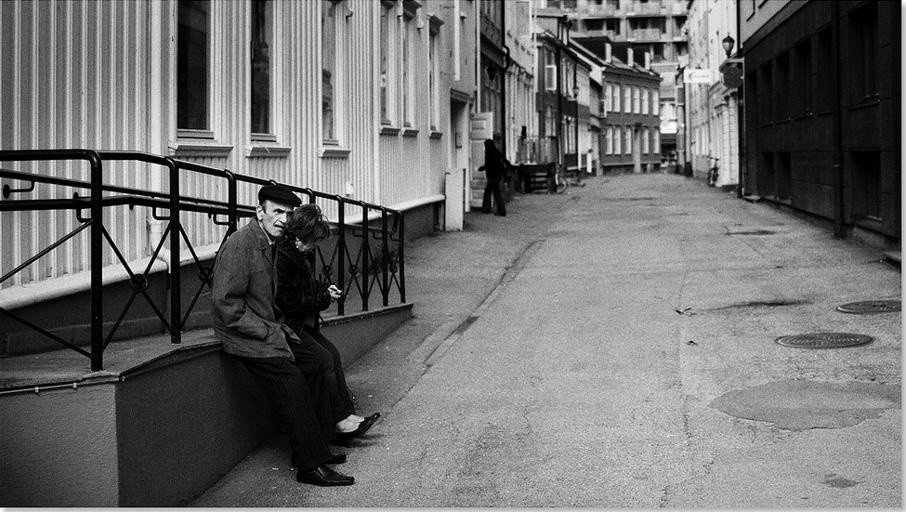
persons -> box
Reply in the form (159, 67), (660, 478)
(272, 205), (380, 447)
(475, 139), (512, 216)
(210, 185), (355, 487)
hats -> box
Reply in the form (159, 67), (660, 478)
(259, 186), (301, 207)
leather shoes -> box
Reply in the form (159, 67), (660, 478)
(297, 465), (354, 486)
(291, 450), (346, 467)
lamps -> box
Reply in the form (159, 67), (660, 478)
(722, 32), (745, 64)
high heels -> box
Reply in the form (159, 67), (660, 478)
(336, 413), (380, 446)
